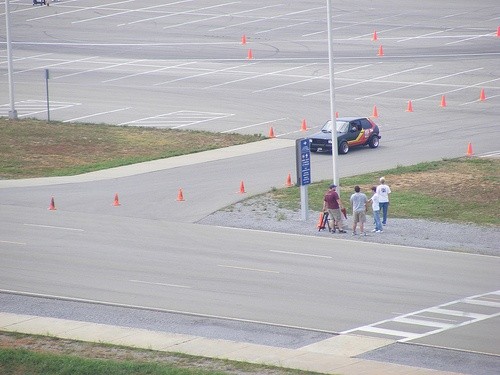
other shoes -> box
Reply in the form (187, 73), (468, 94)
(338, 229), (346, 233)
(331, 230), (335, 234)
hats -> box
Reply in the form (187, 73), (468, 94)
(329, 184), (337, 189)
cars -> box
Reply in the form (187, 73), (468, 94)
(306, 116), (381, 154)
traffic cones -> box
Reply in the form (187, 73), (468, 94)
(496, 25), (500, 37)
(480, 88), (485, 102)
(48, 197), (56, 211)
(335, 112), (338, 118)
(241, 35), (246, 44)
(301, 119), (307, 131)
(373, 31), (377, 40)
(268, 126), (277, 138)
(239, 180), (247, 194)
(378, 45), (383, 56)
(407, 100), (414, 112)
(467, 143), (474, 156)
(112, 192), (121, 206)
(371, 106), (378, 118)
(441, 96), (447, 107)
(285, 173), (294, 186)
(177, 187), (185, 202)
(247, 49), (253, 60)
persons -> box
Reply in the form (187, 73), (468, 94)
(372, 177), (391, 226)
(368, 187), (383, 233)
(351, 125), (356, 130)
(351, 186), (368, 236)
(323, 185), (346, 234)
